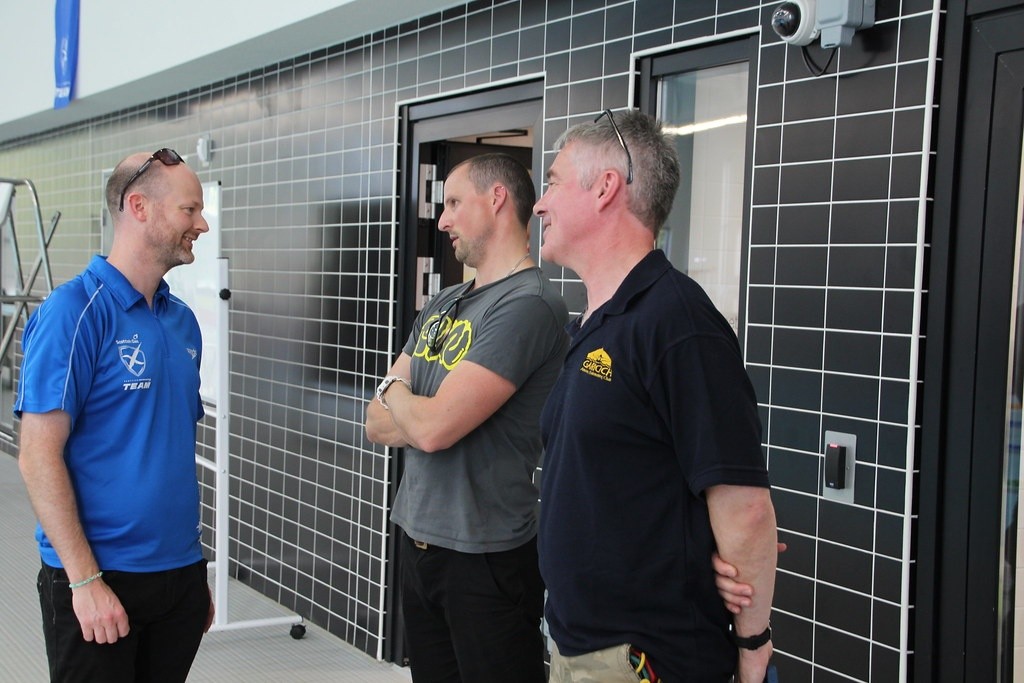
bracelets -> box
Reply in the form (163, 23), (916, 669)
(729, 622), (773, 651)
(68, 571), (103, 588)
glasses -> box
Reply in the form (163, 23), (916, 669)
(119, 148), (186, 212)
(593, 109), (633, 185)
(427, 297), (463, 356)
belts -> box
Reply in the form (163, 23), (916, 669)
(410, 534), (431, 550)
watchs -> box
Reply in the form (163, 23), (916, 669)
(376, 375), (414, 411)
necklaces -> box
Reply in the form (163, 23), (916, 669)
(506, 253), (531, 277)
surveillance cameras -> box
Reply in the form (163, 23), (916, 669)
(771, 0), (819, 46)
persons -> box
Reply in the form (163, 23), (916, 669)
(365, 152), (572, 683)
(533, 111), (787, 683)
(12, 152), (215, 683)
